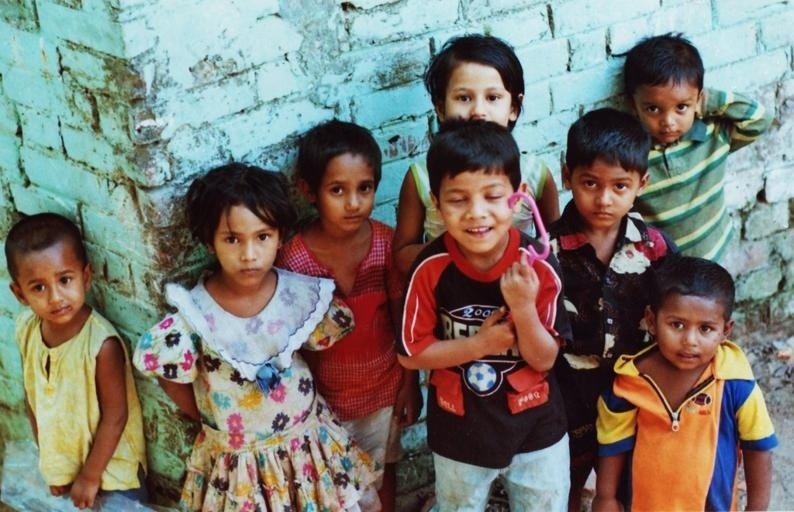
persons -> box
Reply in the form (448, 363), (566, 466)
(4, 212), (151, 511)
(591, 258), (779, 511)
(133, 161), (384, 512)
(546, 105), (677, 512)
(387, 29), (562, 294)
(615, 30), (770, 269)
(392, 116), (574, 512)
(269, 116), (425, 512)
